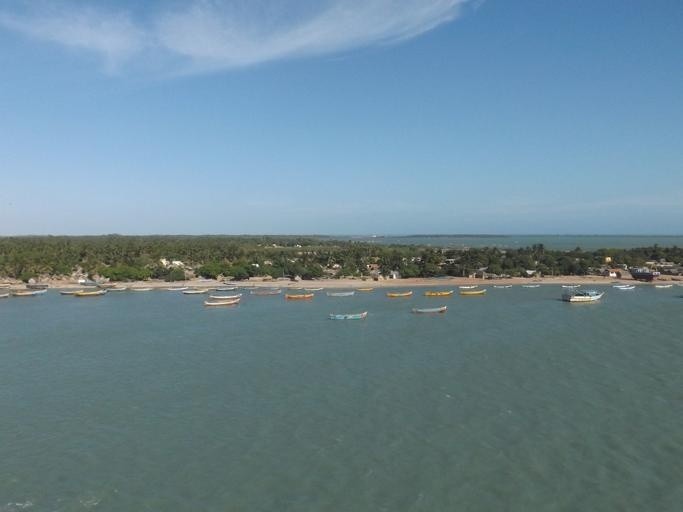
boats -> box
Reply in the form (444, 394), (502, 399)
(493, 285), (512, 289)
(0, 282), (51, 299)
(209, 293), (243, 300)
(655, 284), (673, 288)
(131, 286), (154, 292)
(458, 284), (478, 289)
(385, 290), (413, 297)
(411, 305), (447, 313)
(60, 289), (84, 295)
(328, 311), (369, 320)
(74, 289), (108, 297)
(168, 286), (189, 291)
(326, 290), (356, 297)
(203, 298), (240, 306)
(216, 287), (235, 291)
(284, 293), (314, 300)
(80, 280), (127, 291)
(304, 287), (324, 292)
(612, 284), (636, 291)
(522, 284), (540, 289)
(459, 288), (487, 295)
(356, 286), (373, 292)
(423, 290), (454, 297)
(243, 286), (282, 295)
(560, 283), (581, 288)
(182, 289), (209, 294)
(287, 286), (304, 291)
(561, 289), (605, 303)
